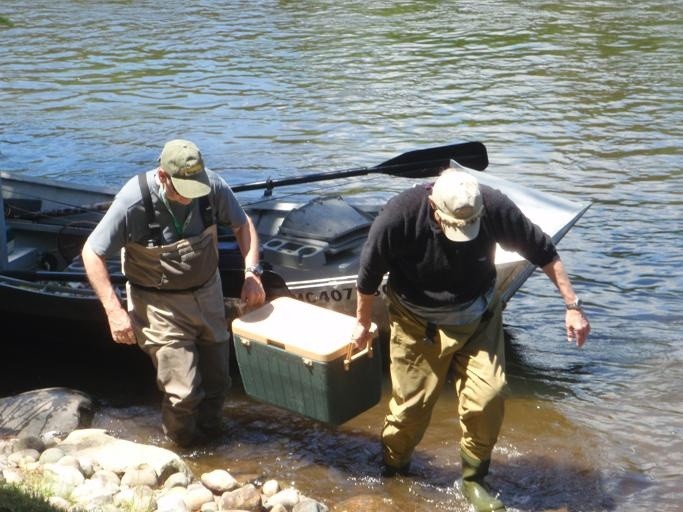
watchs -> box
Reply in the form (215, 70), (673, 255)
(243, 266), (264, 275)
(566, 301), (585, 311)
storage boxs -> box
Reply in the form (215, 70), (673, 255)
(230, 295), (379, 430)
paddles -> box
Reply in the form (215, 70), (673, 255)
(9, 140), (488, 221)
(2, 268), (287, 295)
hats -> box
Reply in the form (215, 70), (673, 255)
(432, 168), (484, 242)
(160, 139), (211, 198)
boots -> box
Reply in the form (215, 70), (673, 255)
(459, 448), (506, 512)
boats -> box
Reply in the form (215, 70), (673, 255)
(0, 159), (594, 332)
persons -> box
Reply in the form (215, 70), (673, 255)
(347, 168), (592, 512)
(82, 138), (266, 446)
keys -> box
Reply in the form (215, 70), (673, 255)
(423, 323), (439, 346)
